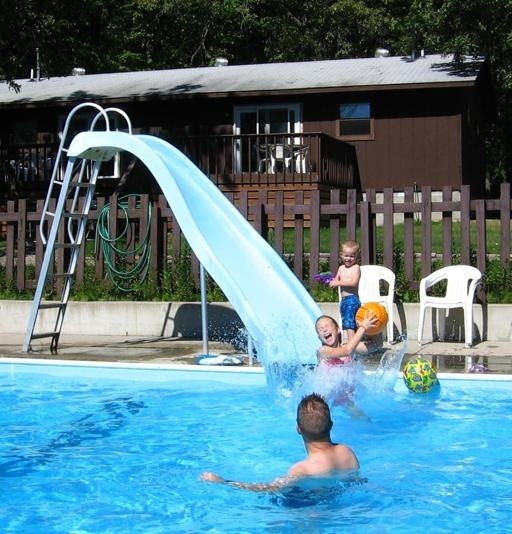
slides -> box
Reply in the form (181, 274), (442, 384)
(66, 131), (324, 386)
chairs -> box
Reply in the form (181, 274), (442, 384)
(338, 264), (405, 344)
(418, 264), (488, 347)
(255, 144), (311, 173)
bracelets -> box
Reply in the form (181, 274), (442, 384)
(359, 325), (366, 332)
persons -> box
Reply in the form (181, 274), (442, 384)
(200, 393), (360, 492)
(328, 240), (361, 344)
(316, 309), (379, 417)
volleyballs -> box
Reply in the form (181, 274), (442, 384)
(403, 356), (438, 394)
(355, 302), (388, 335)
